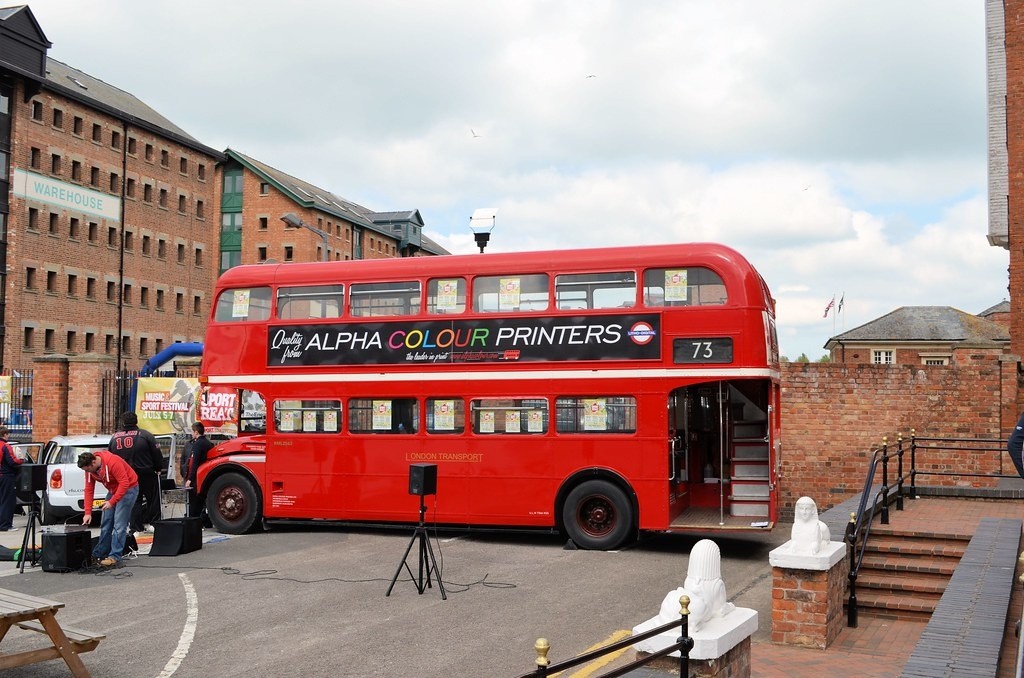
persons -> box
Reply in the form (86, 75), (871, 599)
(107, 411), (163, 533)
(1007, 412), (1023, 478)
(76, 450), (139, 565)
(0, 426), (28, 534)
(691, 394), (717, 482)
(396, 400), (416, 432)
(184, 422), (214, 517)
(179, 431), (195, 516)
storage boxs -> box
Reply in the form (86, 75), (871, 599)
(149, 517), (202, 556)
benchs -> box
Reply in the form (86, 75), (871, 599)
(0, 588), (106, 678)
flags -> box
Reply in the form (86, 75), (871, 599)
(838, 297), (843, 313)
(824, 299), (834, 317)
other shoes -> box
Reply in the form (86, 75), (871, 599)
(100, 557), (117, 565)
(8, 525), (18, 531)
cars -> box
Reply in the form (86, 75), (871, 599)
(2, 407), (33, 434)
(3, 432), (177, 526)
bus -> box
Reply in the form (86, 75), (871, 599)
(191, 240), (783, 543)
